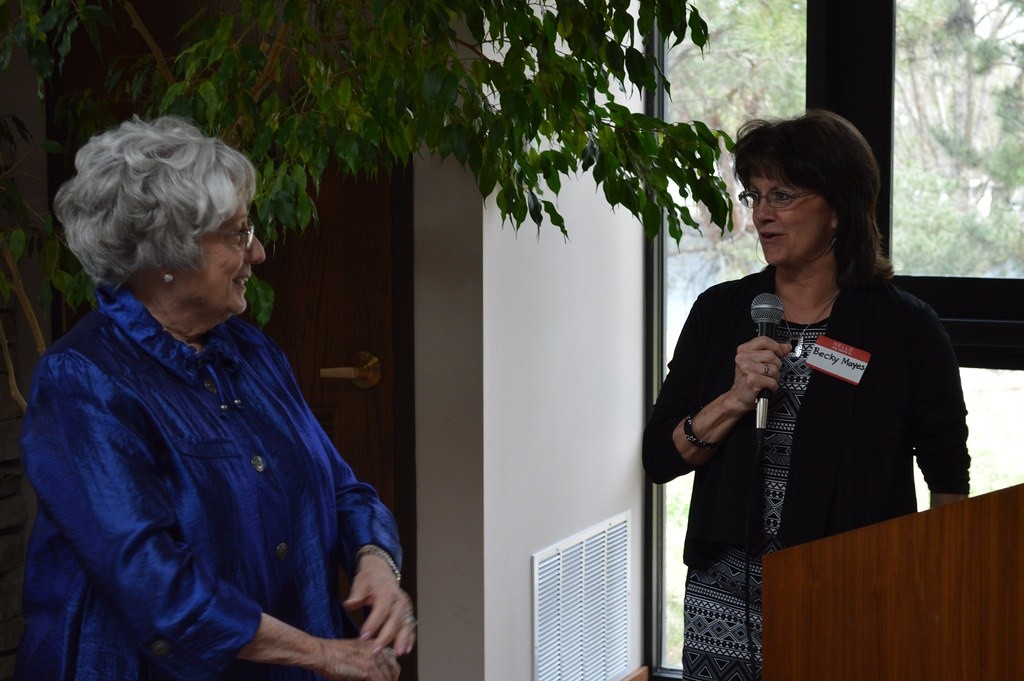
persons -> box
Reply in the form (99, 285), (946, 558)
(12, 113), (420, 681)
(642, 109), (970, 681)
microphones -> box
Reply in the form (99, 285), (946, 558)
(751, 293), (785, 445)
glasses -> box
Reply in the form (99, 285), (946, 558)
(737, 188), (819, 209)
(210, 216), (256, 251)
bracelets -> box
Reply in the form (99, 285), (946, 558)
(355, 546), (402, 588)
(683, 405), (727, 449)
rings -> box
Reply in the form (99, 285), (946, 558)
(763, 362), (770, 377)
(401, 612), (416, 629)
